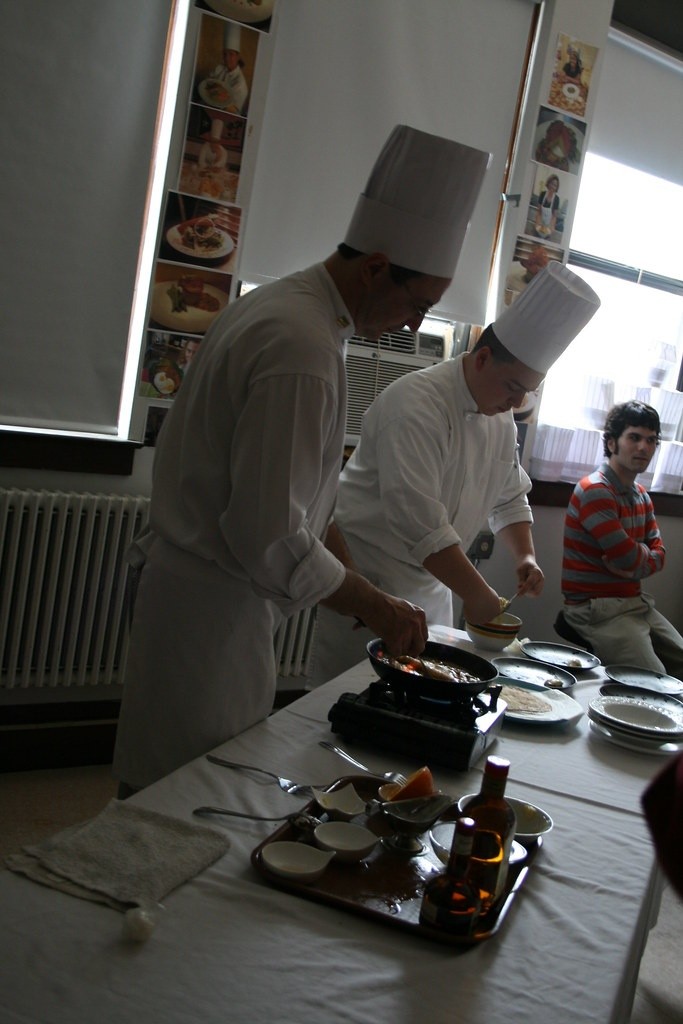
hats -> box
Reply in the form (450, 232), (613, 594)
(344, 124), (490, 279)
(492, 259), (593, 375)
(223, 21), (240, 53)
(567, 46), (579, 59)
(210, 120), (224, 141)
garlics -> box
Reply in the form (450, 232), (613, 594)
(123, 910), (155, 943)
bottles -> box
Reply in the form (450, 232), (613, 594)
(447, 755), (517, 925)
(419, 817), (479, 934)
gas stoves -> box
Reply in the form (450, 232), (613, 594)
(328, 677), (507, 771)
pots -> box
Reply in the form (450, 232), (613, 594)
(366, 637), (499, 697)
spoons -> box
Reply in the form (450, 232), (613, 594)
(193, 806), (323, 832)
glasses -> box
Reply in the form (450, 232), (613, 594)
(387, 263), (432, 320)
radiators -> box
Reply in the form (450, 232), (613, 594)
(0, 486), (333, 703)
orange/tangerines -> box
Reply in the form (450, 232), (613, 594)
(387, 767), (435, 802)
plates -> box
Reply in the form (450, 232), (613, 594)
(166, 224), (234, 258)
(532, 120), (585, 175)
(605, 664), (683, 695)
(151, 281), (229, 333)
(520, 641), (601, 671)
(563, 82), (579, 97)
(497, 676), (584, 723)
(205, 0), (273, 22)
(587, 697), (683, 756)
(491, 657), (577, 689)
(198, 78), (235, 107)
(150, 365), (180, 393)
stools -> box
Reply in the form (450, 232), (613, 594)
(556, 611), (594, 655)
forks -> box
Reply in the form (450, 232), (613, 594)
(318, 740), (408, 786)
(205, 754), (325, 794)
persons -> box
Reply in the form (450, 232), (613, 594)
(195, 120), (228, 177)
(305, 259), (600, 692)
(210, 22), (249, 115)
(110, 124), (492, 803)
(532, 174), (559, 240)
(520, 245), (548, 283)
(556, 44), (584, 87)
(563, 401), (683, 678)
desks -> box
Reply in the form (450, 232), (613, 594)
(0, 623), (677, 1024)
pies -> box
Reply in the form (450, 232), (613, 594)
(490, 683), (552, 713)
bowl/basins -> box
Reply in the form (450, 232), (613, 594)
(458, 793), (553, 844)
(379, 793), (453, 829)
(378, 783), (441, 800)
(262, 840), (337, 878)
(429, 821), (528, 867)
(536, 226), (551, 238)
(314, 821), (382, 861)
(465, 612), (522, 651)
